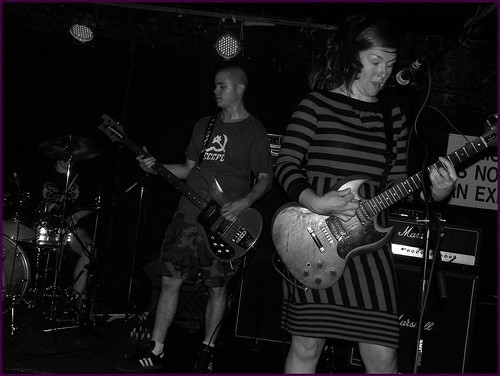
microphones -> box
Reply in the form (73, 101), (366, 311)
(13, 170), (20, 186)
(396, 56), (426, 85)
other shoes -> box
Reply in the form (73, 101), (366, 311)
(117, 340), (164, 372)
(194, 344), (214, 373)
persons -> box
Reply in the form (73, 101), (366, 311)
(273, 12), (457, 374)
(3, 159), (96, 312)
(120, 65), (274, 375)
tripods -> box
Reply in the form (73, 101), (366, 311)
(23, 142), (77, 323)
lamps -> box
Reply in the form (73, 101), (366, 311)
(215, 21), (243, 59)
(70, 10), (96, 42)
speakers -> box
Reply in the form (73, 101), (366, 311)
(235, 163), (293, 347)
(347, 262), (479, 376)
